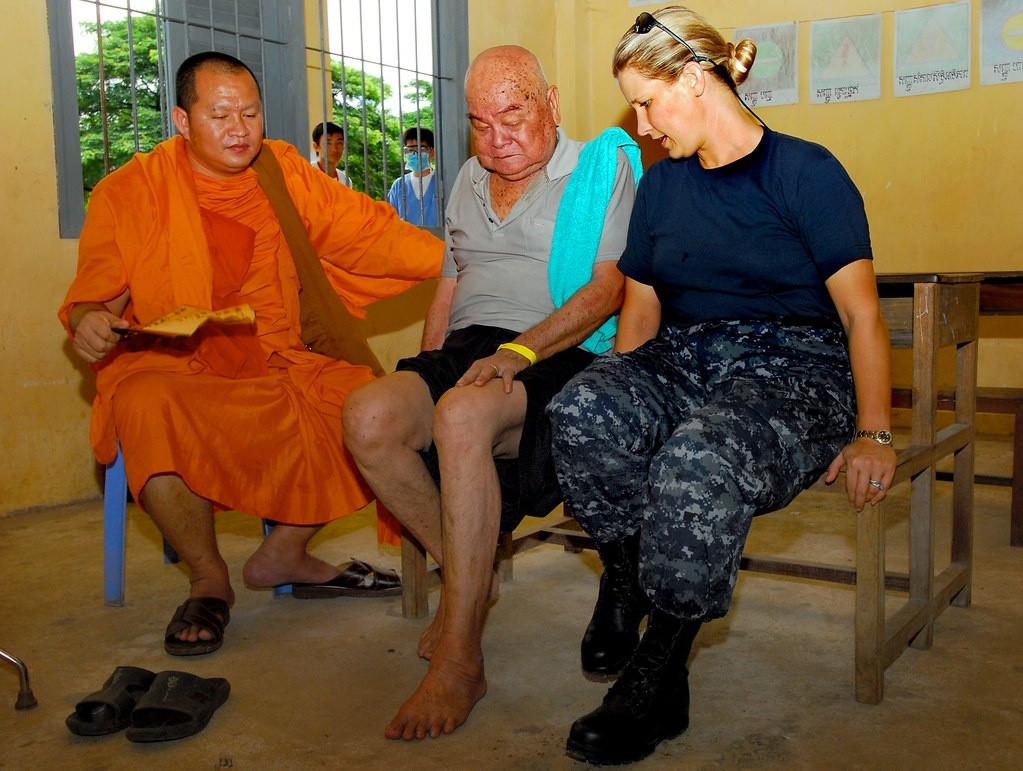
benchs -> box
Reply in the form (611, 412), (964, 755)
(400, 275), (976, 707)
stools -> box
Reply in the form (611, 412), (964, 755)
(104, 435), (293, 607)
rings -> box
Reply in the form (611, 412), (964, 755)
(490, 364), (498, 377)
(870, 480), (882, 489)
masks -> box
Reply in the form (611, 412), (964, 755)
(404, 153), (431, 172)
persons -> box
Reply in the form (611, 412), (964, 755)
(61, 51), (445, 658)
(341, 44), (637, 740)
(389, 126), (440, 229)
(310, 122), (352, 189)
(547, 5), (896, 767)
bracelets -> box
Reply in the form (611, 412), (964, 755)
(497, 343), (537, 365)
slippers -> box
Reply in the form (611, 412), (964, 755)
(126, 670), (231, 741)
(66, 664), (157, 737)
(290, 556), (404, 599)
(164, 597), (230, 655)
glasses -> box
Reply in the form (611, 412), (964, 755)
(634, 12), (699, 64)
(402, 145), (432, 155)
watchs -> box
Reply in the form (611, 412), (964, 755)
(857, 429), (893, 447)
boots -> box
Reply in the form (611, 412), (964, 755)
(566, 605), (702, 766)
(581, 527), (650, 681)
